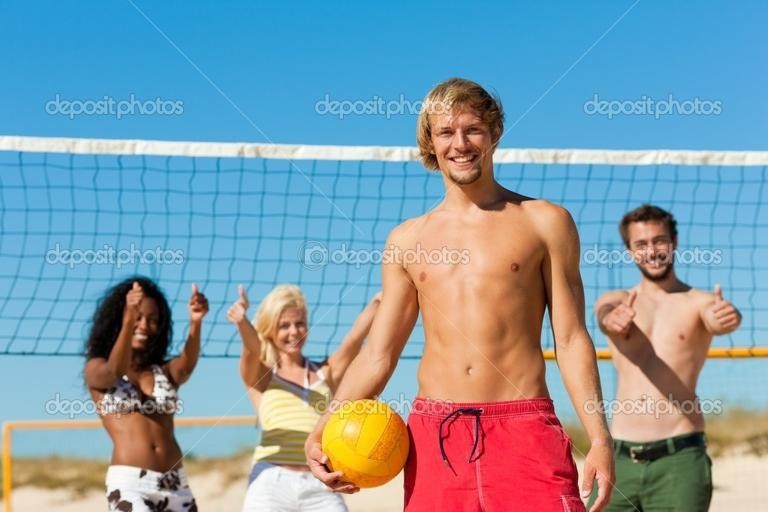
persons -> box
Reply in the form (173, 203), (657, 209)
(584, 201), (743, 512)
(301, 76), (619, 512)
(80, 272), (211, 511)
(224, 283), (385, 512)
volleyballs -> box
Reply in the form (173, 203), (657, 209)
(322, 399), (409, 488)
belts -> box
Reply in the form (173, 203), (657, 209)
(615, 435), (705, 464)
